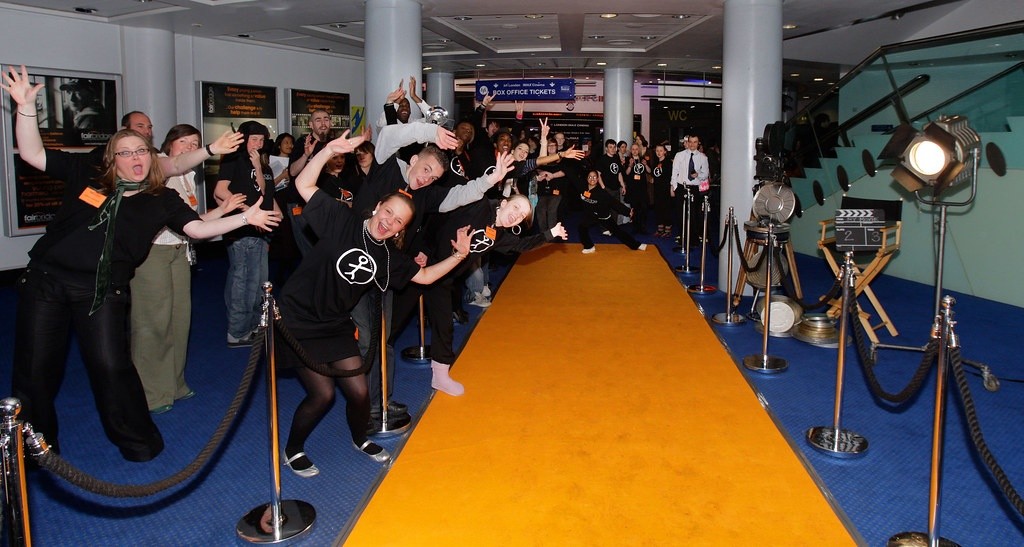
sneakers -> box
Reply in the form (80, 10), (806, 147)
(582, 246), (596, 254)
(226, 326), (259, 348)
(639, 244), (647, 251)
(481, 286), (491, 297)
(468, 293), (492, 307)
(387, 401), (407, 416)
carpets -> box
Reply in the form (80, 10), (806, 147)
(340, 236), (859, 547)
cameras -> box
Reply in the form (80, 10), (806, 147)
(754, 121), (796, 183)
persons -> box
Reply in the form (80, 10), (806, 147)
(552, 134), (721, 254)
(0, 64), (568, 476)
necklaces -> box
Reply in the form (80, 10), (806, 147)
(363, 219), (388, 245)
(362, 220), (390, 292)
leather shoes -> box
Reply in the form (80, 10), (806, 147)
(282, 447), (319, 479)
(352, 437), (390, 463)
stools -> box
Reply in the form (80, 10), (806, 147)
(732, 230), (803, 309)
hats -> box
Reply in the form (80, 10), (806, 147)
(60, 79), (97, 93)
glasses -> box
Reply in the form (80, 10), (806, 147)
(115, 148), (150, 158)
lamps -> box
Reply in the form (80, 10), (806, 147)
(877, 113), (982, 207)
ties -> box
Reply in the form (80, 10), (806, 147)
(688, 153), (695, 180)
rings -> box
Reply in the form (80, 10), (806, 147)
(348, 140), (351, 143)
(350, 142), (353, 145)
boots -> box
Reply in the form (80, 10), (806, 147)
(654, 224), (673, 238)
(430, 360), (464, 396)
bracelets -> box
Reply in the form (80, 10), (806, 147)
(453, 252), (466, 260)
(16, 111), (37, 117)
(539, 138), (547, 145)
(241, 213), (248, 226)
(557, 151), (563, 159)
(205, 143), (216, 157)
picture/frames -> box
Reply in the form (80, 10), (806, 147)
(1, 63), (125, 238)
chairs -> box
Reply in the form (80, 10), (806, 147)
(818, 194), (904, 344)
